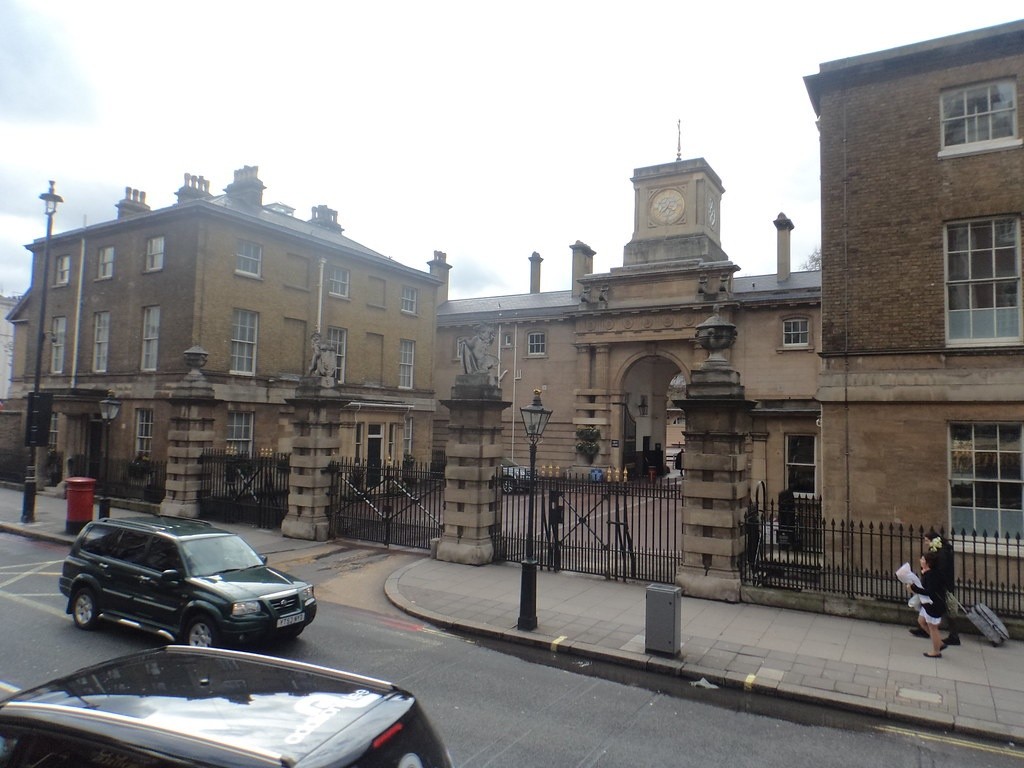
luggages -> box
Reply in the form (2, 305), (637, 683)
(946, 590), (1010, 647)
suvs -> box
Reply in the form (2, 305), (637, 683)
(58, 514), (319, 649)
(0, 645), (453, 768)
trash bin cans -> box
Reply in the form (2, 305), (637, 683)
(64, 475), (96, 535)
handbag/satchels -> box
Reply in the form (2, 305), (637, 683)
(945, 590), (958, 620)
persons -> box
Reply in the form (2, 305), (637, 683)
(906, 532), (961, 658)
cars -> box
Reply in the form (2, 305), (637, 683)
(499, 458), (540, 495)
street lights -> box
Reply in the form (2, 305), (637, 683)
(19, 179), (65, 525)
(517, 388), (555, 630)
(98, 389), (122, 522)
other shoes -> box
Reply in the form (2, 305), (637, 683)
(923, 653), (942, 658)
(909, 628), (930, 638)
(942, 635), (960, 645)
(940, 644), (947, 651)
